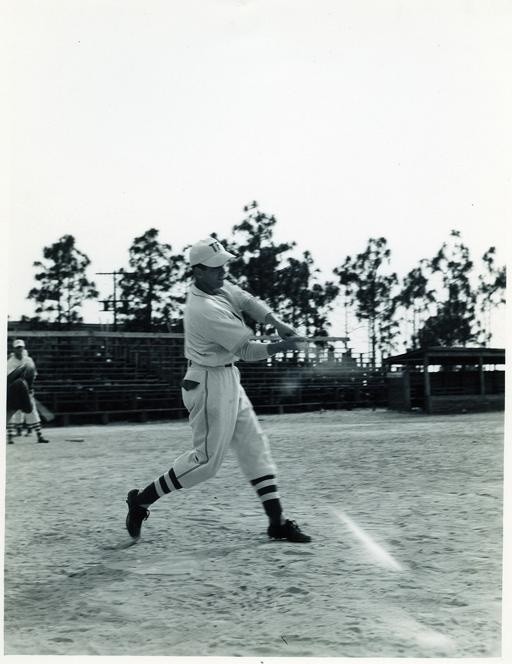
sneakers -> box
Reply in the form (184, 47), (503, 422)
(7, 432), (48, 444)
(267, 519), (310, 542)
(126, 489), (149, 537)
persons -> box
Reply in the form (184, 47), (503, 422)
(13, 349), (33, 437)
(7, 337), (50, 444)
(123, 235), (315, 544)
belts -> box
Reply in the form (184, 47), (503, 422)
(224, 363), (231, 367)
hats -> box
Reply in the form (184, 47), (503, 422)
(190, 236), (236, 268)
(13, 340), (24, 347)
